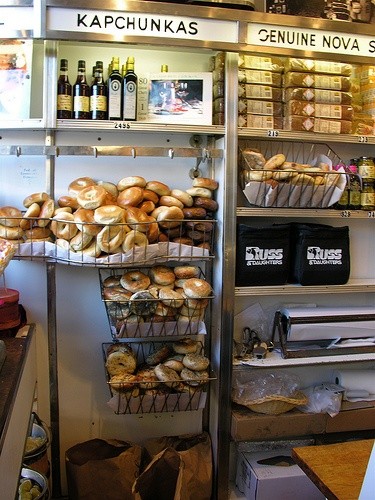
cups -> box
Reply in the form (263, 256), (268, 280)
(0, 287), (21, 330)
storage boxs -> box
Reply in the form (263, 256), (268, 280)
(230, 405), (326, 442)
(326, 407), (375, 433)
(235, 451), (326, 500)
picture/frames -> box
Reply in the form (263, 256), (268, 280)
(147, 71), (212, 125)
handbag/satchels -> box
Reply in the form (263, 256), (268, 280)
(236, 223), (290, 286)
(287, 221), (350, 284)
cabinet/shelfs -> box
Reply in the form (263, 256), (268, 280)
(0, 0), (375, 500)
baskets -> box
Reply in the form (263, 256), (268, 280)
(231, 387), (307, 414)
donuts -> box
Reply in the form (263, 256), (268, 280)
(0, 176), (218, 260)
(103, 265), (211, 331)
(105, 339), (209, 398)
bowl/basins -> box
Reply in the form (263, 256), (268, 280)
(16, 467), (50, 500)
(22, 417), (51, 463)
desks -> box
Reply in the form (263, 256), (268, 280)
(0, 324), (35, 500)
(291, 439), (374, 500)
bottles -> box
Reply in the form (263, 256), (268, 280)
(72, 60), (90, 120)
(57, 59), (73, 119)
(162, 65), (167, 73)
(90, 56), (138, 121)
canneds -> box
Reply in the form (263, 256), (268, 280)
(333, 156), (375, 211)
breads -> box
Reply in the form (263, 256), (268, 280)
(236, 54), (375, 138)
(24, 437), (41, 452)
(239, 147), (341, 187)
(17, 478), (41, 500)
